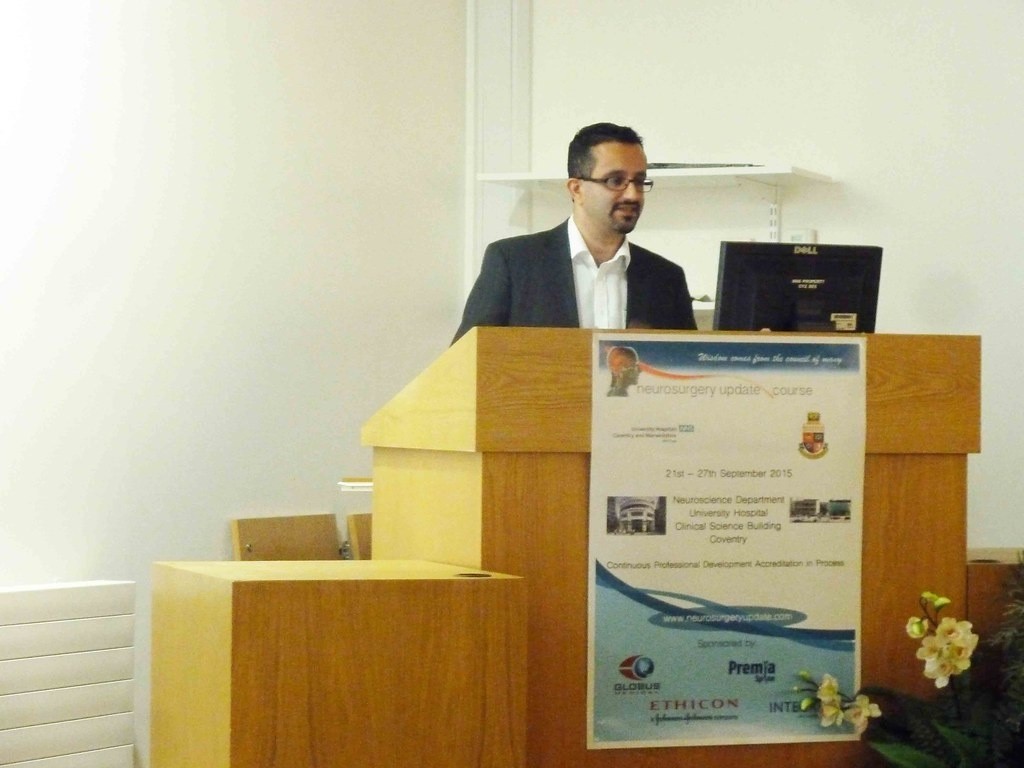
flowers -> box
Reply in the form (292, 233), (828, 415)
(794, 586), (1024, 768)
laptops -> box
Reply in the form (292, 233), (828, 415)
(710, 239), (882, 334)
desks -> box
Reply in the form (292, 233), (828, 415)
(150, 558), (530, 768)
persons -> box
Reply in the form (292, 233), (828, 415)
(450, 122), (698, 346)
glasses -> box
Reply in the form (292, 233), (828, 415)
(575, 176), (654, 192)
